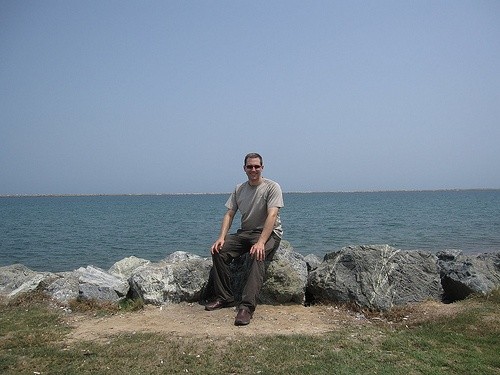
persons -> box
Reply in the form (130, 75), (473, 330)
(204, 153), (285, 325)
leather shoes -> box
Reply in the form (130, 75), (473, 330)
(236, 309), (252, 325)
(205, 301), (225, 311)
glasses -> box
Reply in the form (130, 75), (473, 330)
(246, 165), (261, 169)
